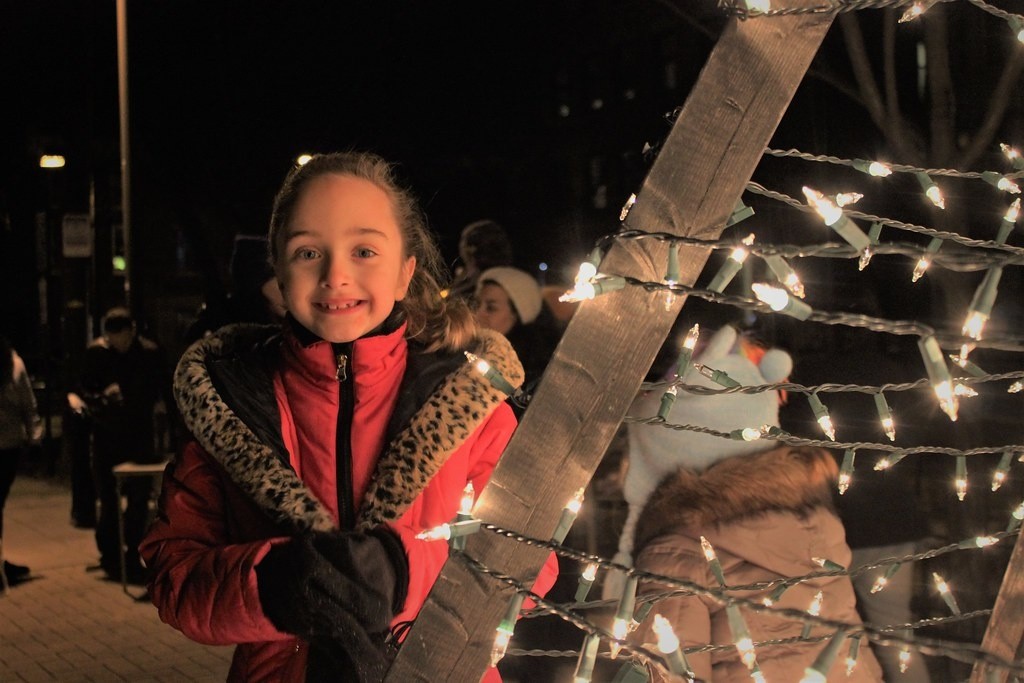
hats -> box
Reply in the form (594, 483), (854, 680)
(473, 267), (543, 325)
(601, 327), (793, 600)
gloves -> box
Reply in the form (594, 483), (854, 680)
(257, 527), (409, 683)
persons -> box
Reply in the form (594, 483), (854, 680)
(452, 213), (569, 408)
(601, 331), (886, 683)
(0, 329), (40, 580)
(165, 285), (243, 456)
(58, 309), (165, 583)
(134, 150), (559, 683)
(785, 285), (932, 682)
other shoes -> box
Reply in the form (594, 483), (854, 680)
(0, 560), (30, 588)
